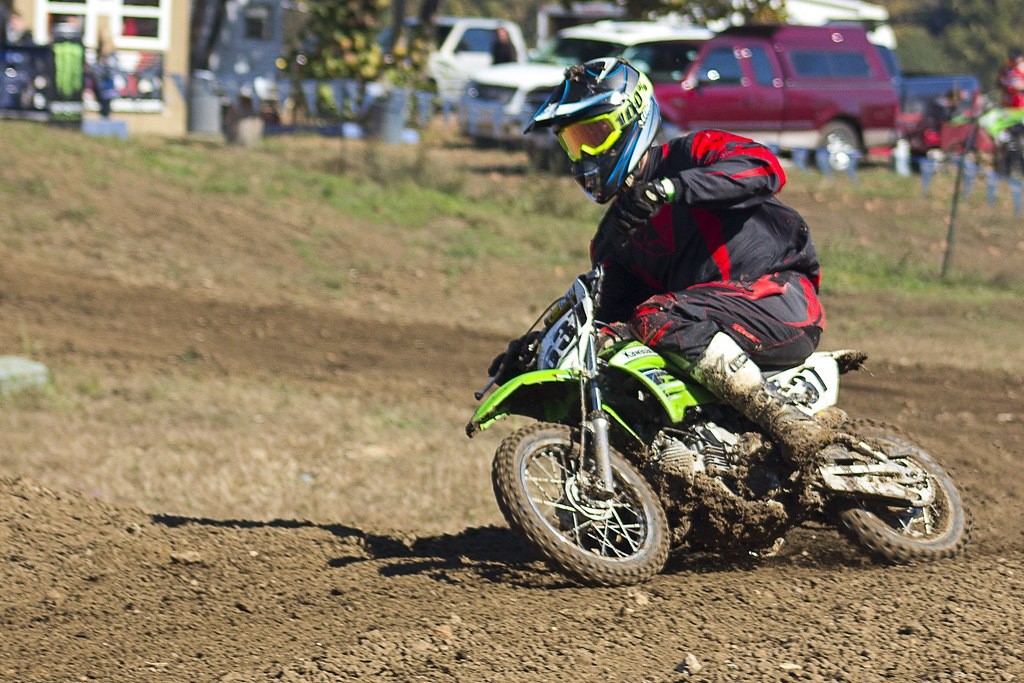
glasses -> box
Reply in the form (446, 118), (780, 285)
(554, 113), (621, 161)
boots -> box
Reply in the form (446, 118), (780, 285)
(685, 331), (836, 459)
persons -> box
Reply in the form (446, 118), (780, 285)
(488, 58), (834, 462)
(909, 87), (962, 174)
(43, 20), (85, 136)
(90, 46), (118, 116)
(995, 57), (1024, 177)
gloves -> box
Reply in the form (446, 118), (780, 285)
(609, 176), (676, 233)
(488, 332), (540, 387)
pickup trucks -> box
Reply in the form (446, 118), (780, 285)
(611, 25), (905, 176)
(872, 43), (981, 116)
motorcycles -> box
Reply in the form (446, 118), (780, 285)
(463, 191), (976, 587)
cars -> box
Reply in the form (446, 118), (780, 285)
(452, 22), (715, 143)
(373, 16), (526, 114)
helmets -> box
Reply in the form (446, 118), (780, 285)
(523, 57), (662, 205)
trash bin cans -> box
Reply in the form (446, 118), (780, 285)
(367, 88), (404, 140)
(185, 68), (220, 133)
